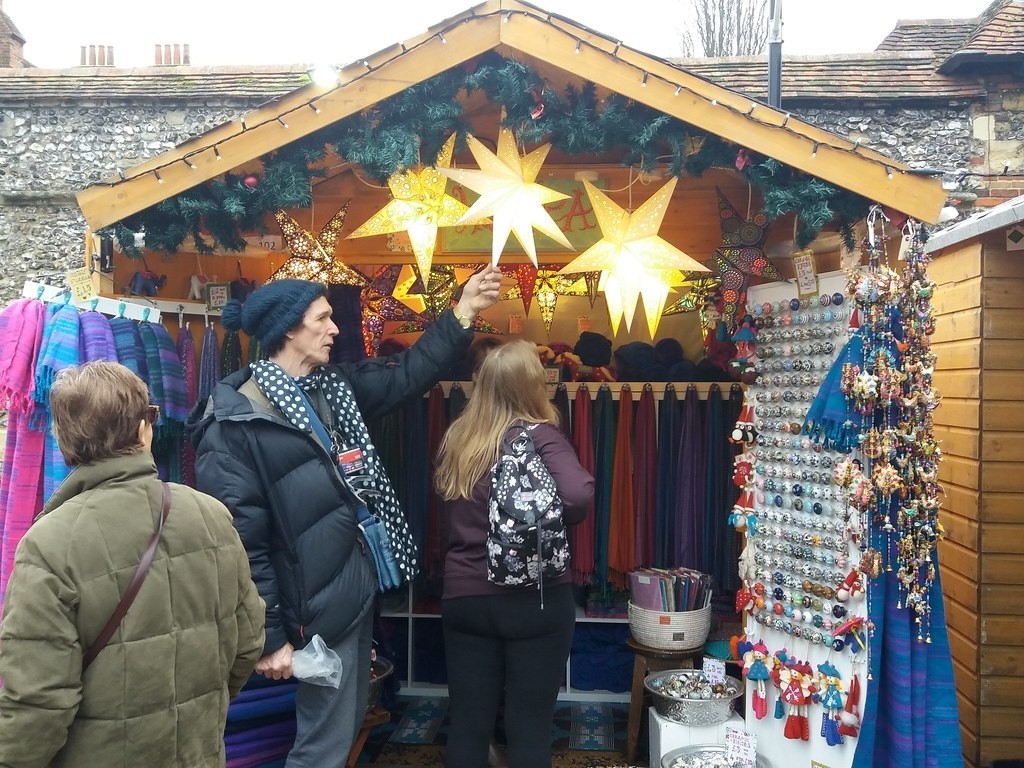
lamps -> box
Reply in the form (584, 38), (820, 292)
(259, 102), (792, 359)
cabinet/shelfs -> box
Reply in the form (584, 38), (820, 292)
(377, 578), (633, 704)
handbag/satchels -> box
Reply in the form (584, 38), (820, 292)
(359, 503), (402, 594)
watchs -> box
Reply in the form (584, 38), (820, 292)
(453, 306), (476, 330)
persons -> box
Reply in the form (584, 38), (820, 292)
(434, 337), (595, 768)
(0, 360), (266, 768)
(184, 262), (503, 768)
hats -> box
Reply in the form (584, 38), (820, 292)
(572, 332), (721, 382)
(221, 278), (327, 353)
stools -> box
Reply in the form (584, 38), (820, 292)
(702, 654), (746, 720)
(348, 705), (390, 768)
(624, 635), (712, 765)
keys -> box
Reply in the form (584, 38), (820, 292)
(331, 451), (339, 470)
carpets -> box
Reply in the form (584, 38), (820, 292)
(386, 697), (625, 750)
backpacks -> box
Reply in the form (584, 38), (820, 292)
(486, 425), (571, 610)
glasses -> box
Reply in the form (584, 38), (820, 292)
(145, 405), (158, 425)
